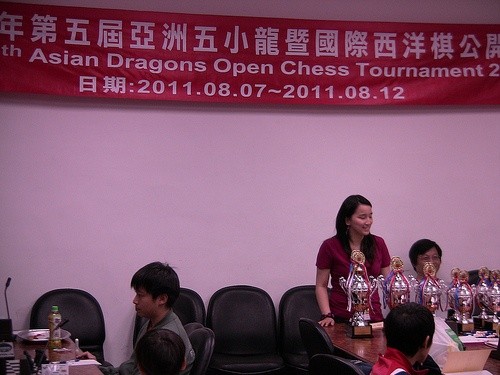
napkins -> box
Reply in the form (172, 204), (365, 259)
(52, 360), (101, 367)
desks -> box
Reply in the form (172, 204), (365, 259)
(322, 322), (500, 375)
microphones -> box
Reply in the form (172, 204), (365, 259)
(35, 318), (68, 370)
(4, 278), (11, 320)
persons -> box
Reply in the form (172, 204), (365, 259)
(134, 328), (186, 375)
(315, 195), (392, 326)
(369, 302), (435, 375)
(76, 262), (196, 375)
(408, 239), (453, 322)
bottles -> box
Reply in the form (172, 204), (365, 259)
(48, 306), (62, 349)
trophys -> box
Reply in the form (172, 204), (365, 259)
(378, 256), (500, 331)
(339, 249), (378, 339)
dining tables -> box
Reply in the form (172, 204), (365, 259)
(0, 334), (101, 375)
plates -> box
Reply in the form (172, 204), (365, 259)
(17, 329), (71, 341)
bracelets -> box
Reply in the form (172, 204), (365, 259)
(320, 313), (334, 318)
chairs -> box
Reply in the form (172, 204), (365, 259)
(29, 285), (372, 375)
